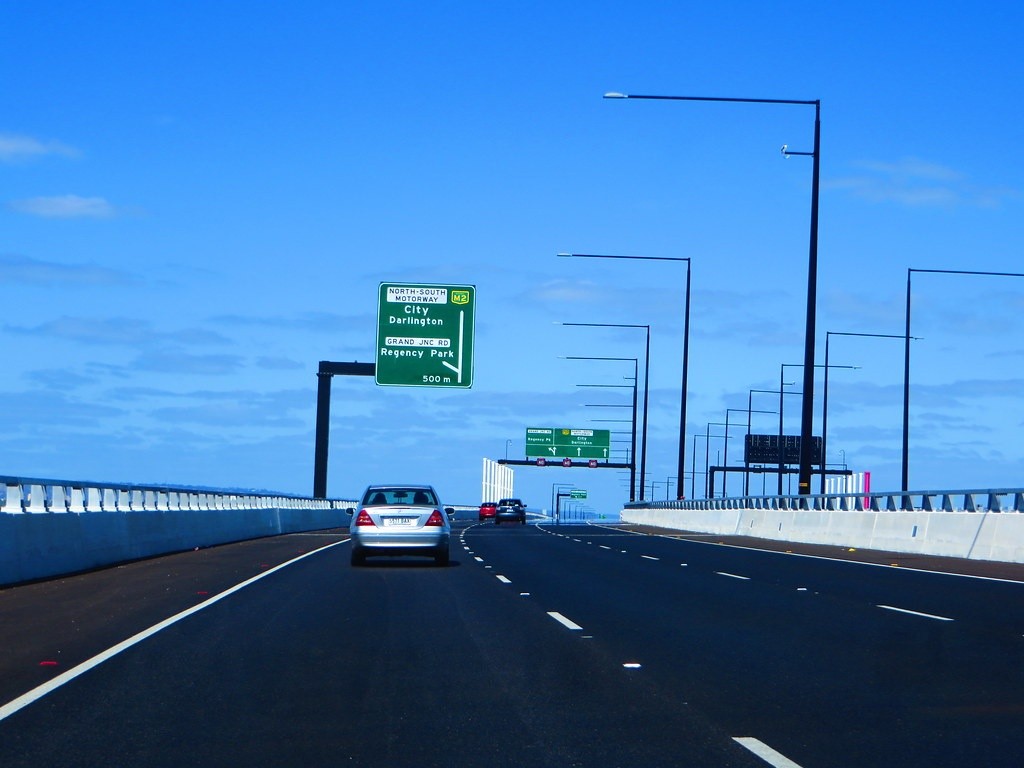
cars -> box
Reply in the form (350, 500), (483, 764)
(494, 498), (528, 525)
(345, 485), (456, 569)
(478, 502), (499, 521)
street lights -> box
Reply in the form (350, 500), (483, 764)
(569, 383), (637, 501)
(778, 363), (862, 483)
(557, 356), (639, 500)
(604, 92), (820, 510)
(558, 252), (692, 499)
(505, 439), (512, 466)
(820, 330), (925, 501)
(552, 320), (650, 499)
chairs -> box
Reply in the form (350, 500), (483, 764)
(372, 494), (387, 505)
(414, 494), (428, 504)
